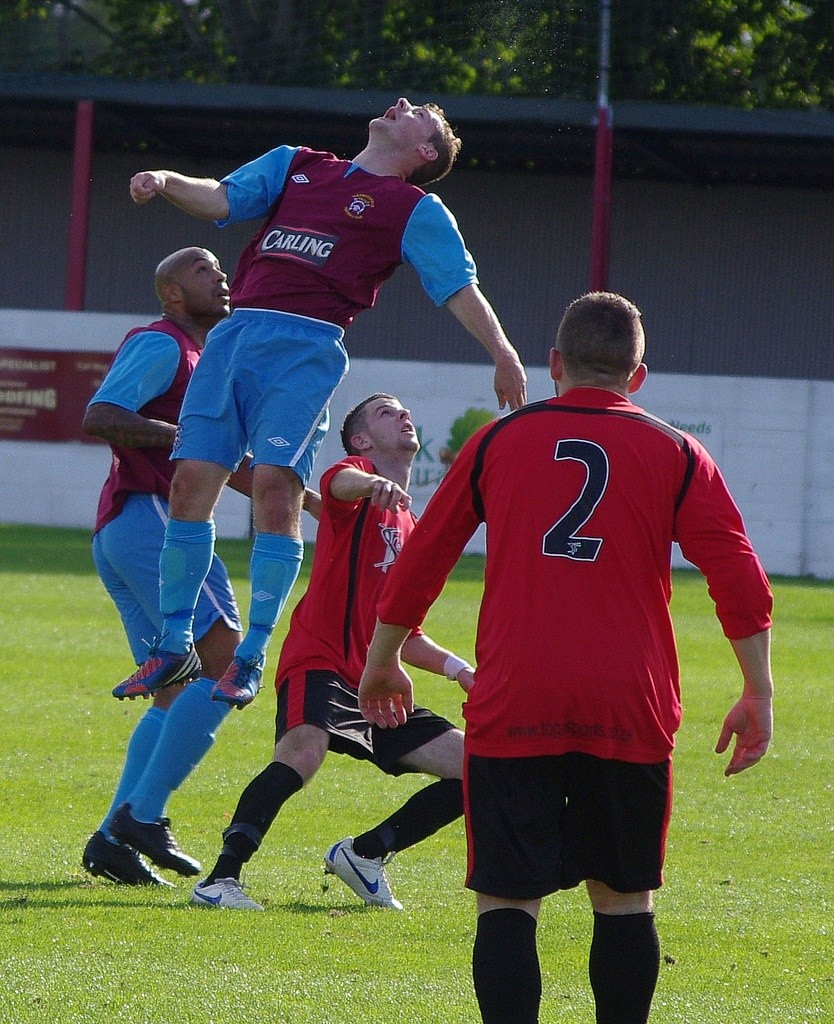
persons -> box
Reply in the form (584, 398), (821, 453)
(358, 292), (774, 1024)
(193, 394), (477, 911)
(112, 97), (527, 711)
(81, 247), (322, 889)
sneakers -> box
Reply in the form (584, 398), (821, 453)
(82, 832), (177, 889)
(324, 836), (404, 910)
(210, 653), (266, 709)
(111, 630), (203, 701)
(108, 803), (201, 877)
(192, 873), (266, 911)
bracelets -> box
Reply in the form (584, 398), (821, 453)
(444, 656), (471, 682)
